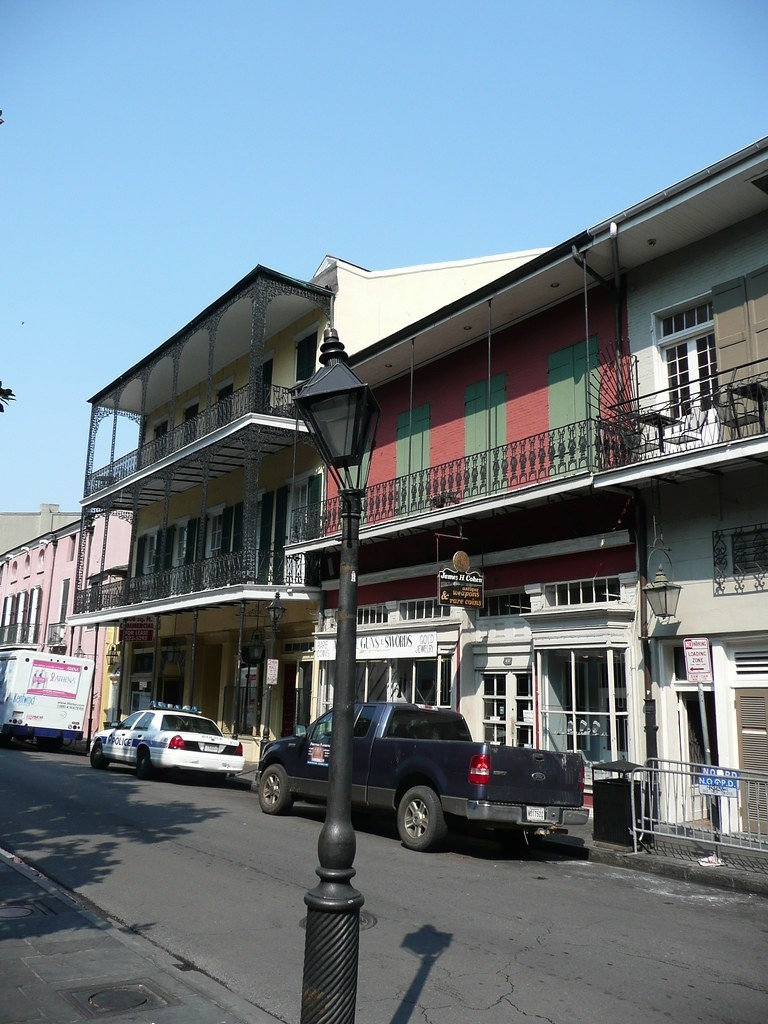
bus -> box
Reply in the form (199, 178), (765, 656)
(0, 650), (95, 753)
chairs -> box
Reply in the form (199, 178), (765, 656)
(395, 724), (424, 739)
(617, 398), (760, 462)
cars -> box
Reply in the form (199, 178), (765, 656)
(88, 701), (245, 786)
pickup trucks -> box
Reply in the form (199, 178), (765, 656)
(254, 701), (590, 853)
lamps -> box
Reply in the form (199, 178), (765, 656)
(166, 614), (180, 664)
(249, 601), (263, 660)
(74, 626), (84, 657)
(643, 478), (682, 619)
(106, 621), (118, 665)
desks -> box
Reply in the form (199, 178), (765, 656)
(733, 383), (768, 433)
(638, 414), (684, 452)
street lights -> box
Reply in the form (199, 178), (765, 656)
(250, 590), (288, 796)
(292, 321), (381, 1024)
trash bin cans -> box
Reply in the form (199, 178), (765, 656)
(591, 758), (655, 847)
